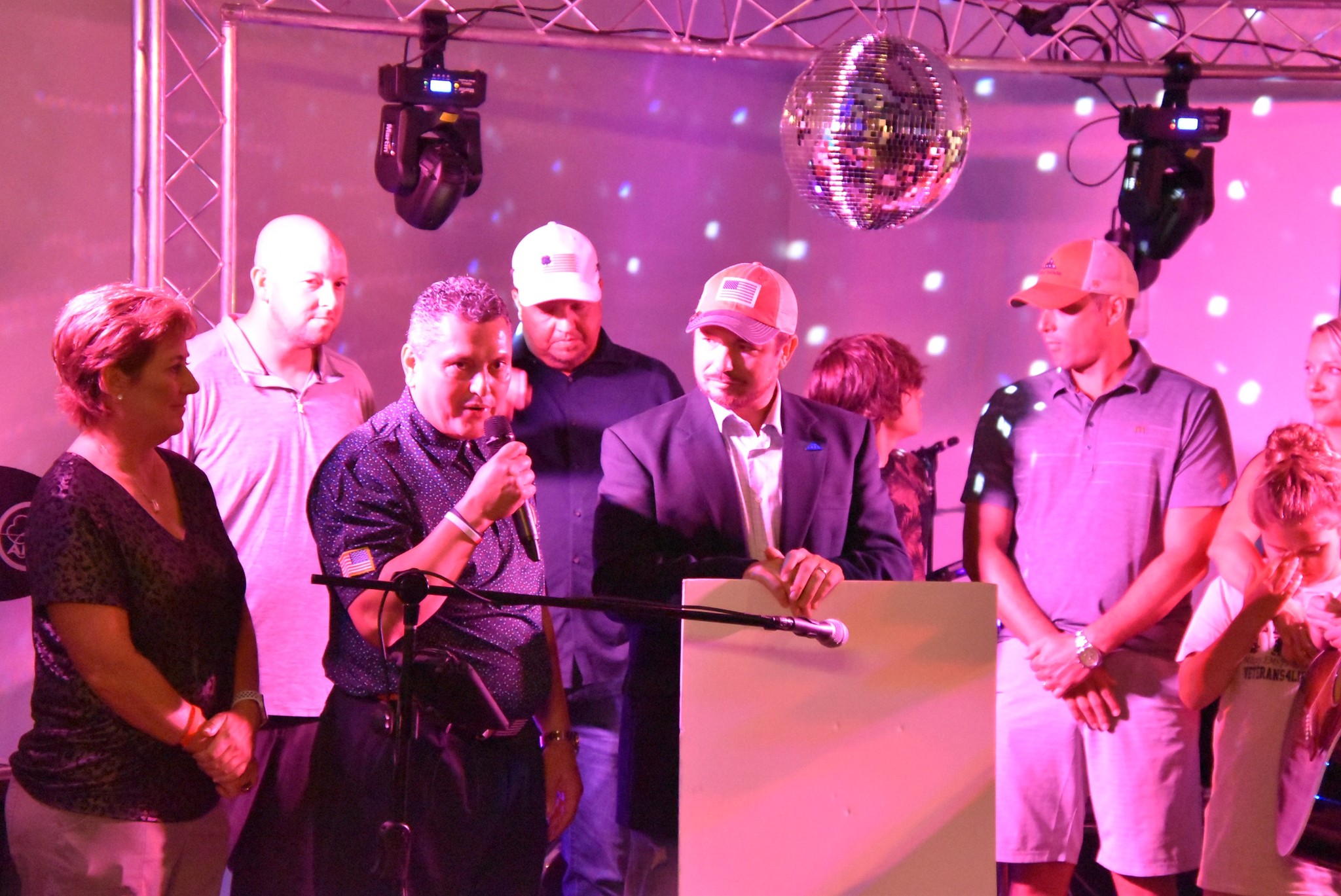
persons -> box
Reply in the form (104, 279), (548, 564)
(1173, 422), (1341, 896)
(802, 331), (934, 581)
(305, 276), (585, 895)
(159, 214), (377, 895)
(960, 239), (1237, 896)
(5, 284), (269, 895)
(592, 260), (913, 896)
(511, 221), (688, 896)
(1207, 316), (1341, 668)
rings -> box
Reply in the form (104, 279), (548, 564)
(816, 566), (828, 577)
(1043, 682), (1050, 692)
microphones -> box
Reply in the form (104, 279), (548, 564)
(484, 414), (544, 562)
(772, 616), (849, 648)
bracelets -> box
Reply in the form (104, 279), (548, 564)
(539, 730), (581, 755)
(443, 507), (484, 544)
(231, 691), (268, 728)
(180, 705), (202, 753)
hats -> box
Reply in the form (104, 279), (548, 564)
(1007, 238), (1140, 312)
(686, 261), (801, 345)
(512, 222), (603, 309)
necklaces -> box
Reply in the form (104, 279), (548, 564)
(94, 436), (161, 512)
(261, 363), (314, 413)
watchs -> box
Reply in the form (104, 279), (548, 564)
(1075, 629), (1103, 670)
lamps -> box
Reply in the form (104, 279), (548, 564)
(374, 9), (486, 230)
(779, 0), (970, 232)
(1119, 53), (1230, 259)
